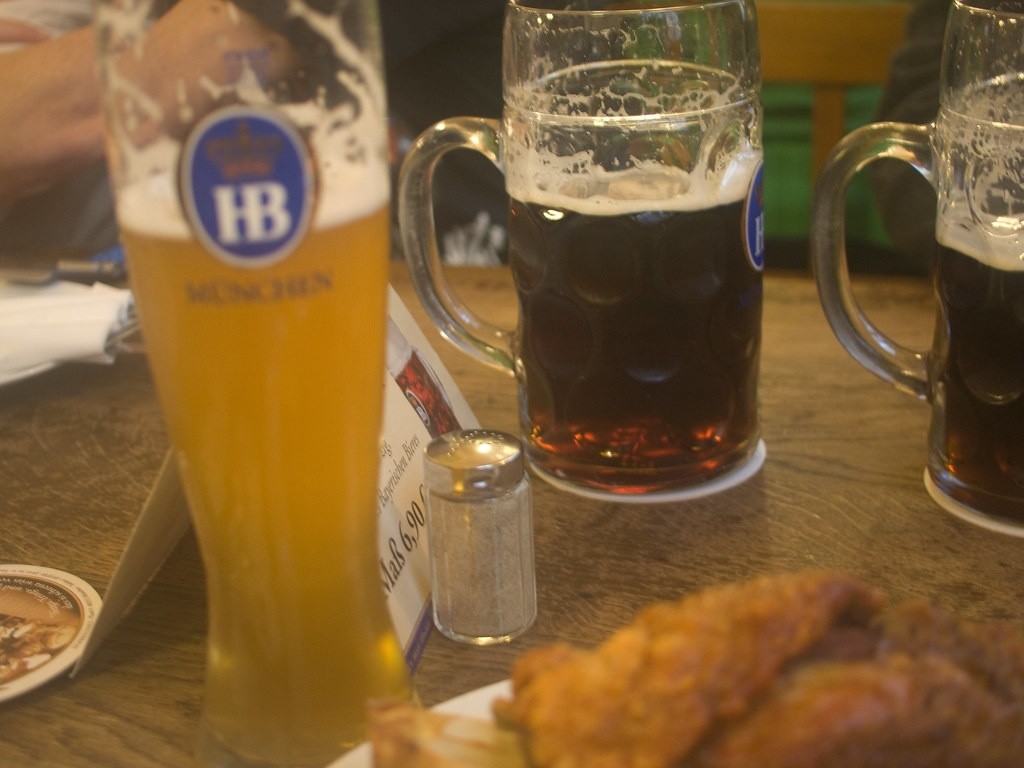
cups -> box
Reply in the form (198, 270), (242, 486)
(96, 0), (424, 768)
(397, 0), (765, 495)
(811, 0), (1024, 537)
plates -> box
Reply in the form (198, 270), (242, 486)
(325, 679), (515, 768)
(0, 321), (140, 388)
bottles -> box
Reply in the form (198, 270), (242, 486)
(423, 429), (537, 645)
